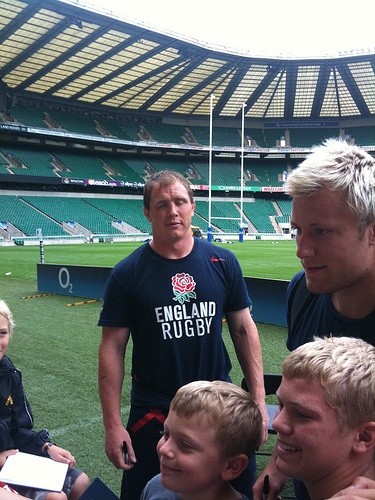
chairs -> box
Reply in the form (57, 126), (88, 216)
(241, 375), (283, 456)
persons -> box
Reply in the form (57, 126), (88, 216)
(0, 299), (90, 500)
(251, 137), (375, 500)
(97, 171), (269, 500)
(140, 380), (269, 500)
(271, 333), (375, 500)
